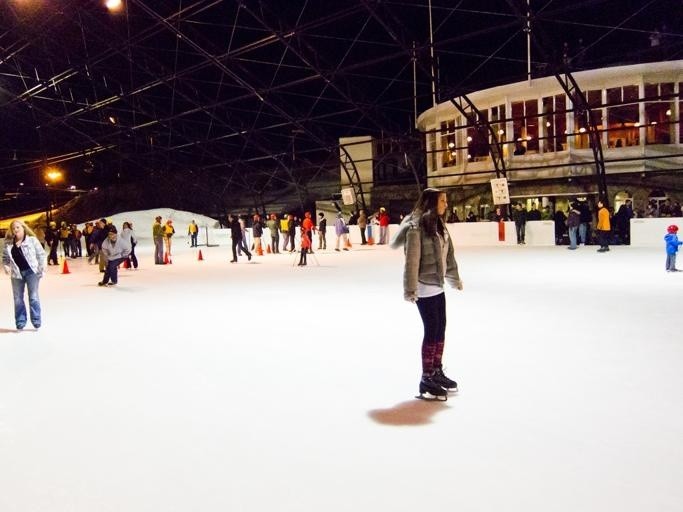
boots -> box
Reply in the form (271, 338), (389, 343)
(420, 364), (456, 396)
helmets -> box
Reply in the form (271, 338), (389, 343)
(50, 222), (76, 227)
(380, 208), (385, 212)
(156, 215), (172, 224)
(301, 226), (306, 232)
(667, 225), (678, 234)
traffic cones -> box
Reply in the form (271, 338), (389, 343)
(267, 243), (271, 255)
(196, 249), (204, 260)
(61, 259), (69, 275)
(256, 243), (262, 256)
(121, 256), (127, 268)
(366, 236), (375, 245)
(347, 238), (351, 247)
(162, 250), (168, 264)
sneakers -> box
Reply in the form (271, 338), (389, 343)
(17, 324), (40, 328)
(98, 281), (117, 286)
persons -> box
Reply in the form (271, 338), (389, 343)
(1, 220), (47, 329)
(514, 141), (527, 155)
(615, 138), (624, 148)
(390, 188), (464, 395)
(446, 197), (683, 252)
(226, 207), (391, 266)
(663, 224), (683, 273)
(187, 219), (198, 247)
(29, 215), (176, 287)
